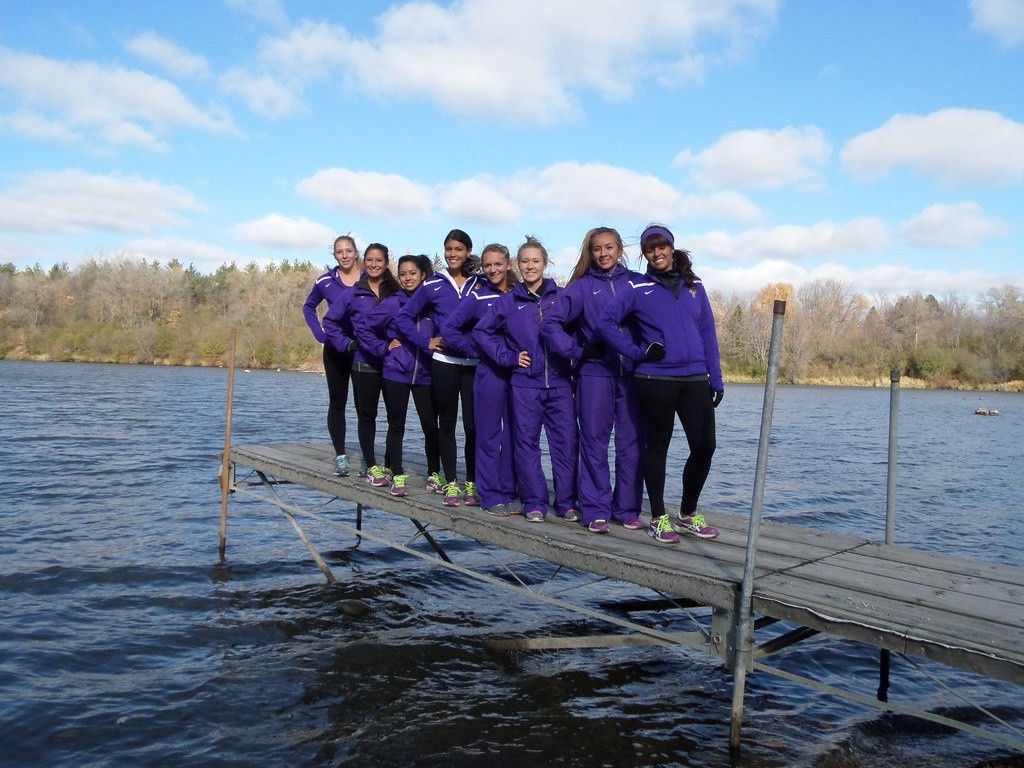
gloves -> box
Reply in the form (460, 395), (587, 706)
(582, 345), (604, 359)
(709, 386), (724, 407)
(646, 341), (666, 362)
(348, 340), (362, 352)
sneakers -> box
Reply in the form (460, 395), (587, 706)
(623, 517), (644, 531)
(380, 464), (393, 479)
(390, 475), (408, 496)
(508, 497), (523, 515)
(366, 466), (392, 487)
(526, 510), (546, 524)
(442, 481), (461, 507)
(588, 520), (613, 532)
(463, 481), (482, 505)
(333, 455), (352, 477)
(648, 515), (683, 544)
(426, 472), (447, 495)
(480, 499), (509, 517)
(564, 509), (581, 521)
(358, 458), (369, 477)
(673, 512), (720, 541)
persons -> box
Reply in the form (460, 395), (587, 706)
(356, 254), (447, 497)
(471, 236), (581, 522)
(303, 237), (367, 477)
(396, 229), (481, 506)
(540, 227), (646, 532)
(322, 242), (402, 486)
(595, 225), (724, 543)
(440, 243), (523, 517)
